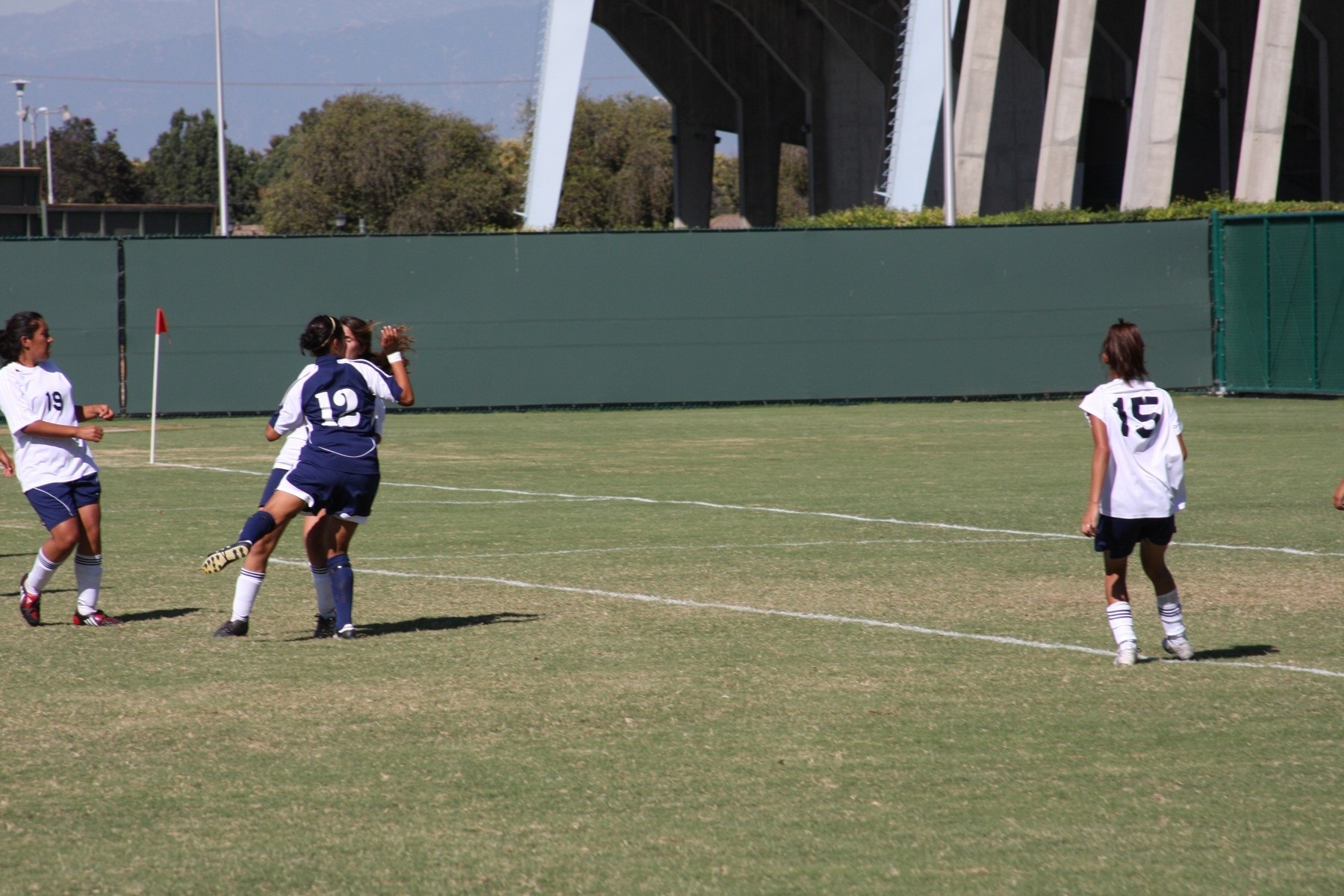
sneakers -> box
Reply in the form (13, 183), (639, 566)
(1112, 647), (1142, 665)
(313, 612), (337, 638)
(330, 629), (357, 639)
(1162, 638), (1195, 659)
(74, 610), (122, 626)
(213, 619), (249, 637)
(19, 573), (41, 627)
(201, 541), (252, 575)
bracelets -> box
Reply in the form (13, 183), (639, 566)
(386, 350), (403, 365)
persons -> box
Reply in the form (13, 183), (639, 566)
(0, 311), (123, 628)
(0, 446), (14, 478)
(199, 314), (414, 641)
(1333, 479), (1344, 510)
(1077, 317), (1195, 667)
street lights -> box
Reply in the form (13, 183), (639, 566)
(15, 103), (74, 205)
(7, 79), (31, 167)
